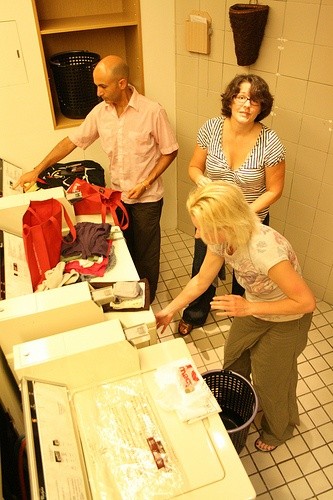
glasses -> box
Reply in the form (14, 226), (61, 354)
(230, 94), (262, 106)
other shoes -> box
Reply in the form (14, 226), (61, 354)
(254, 437), (278, 452)
(179, 321), (193, 335)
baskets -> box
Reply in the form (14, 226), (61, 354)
(199, 369), (258, 455)
(47, 51), (100, 119)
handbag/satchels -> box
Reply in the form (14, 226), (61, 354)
(35, 160), (106, 193)
(66, 177), (130, 230)
(21, 198), (77, 294)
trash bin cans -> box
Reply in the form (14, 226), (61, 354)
(201, 369), (259, 456)
(49, 49), (102, 120)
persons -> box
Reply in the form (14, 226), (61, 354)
(154, 182), (317, 453)
(12, 55), (179, 307)
(177, 74), (287, 335)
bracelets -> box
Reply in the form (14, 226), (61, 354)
(141, 179), (151, 191)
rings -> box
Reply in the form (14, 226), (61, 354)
(224, 306), (228, 311)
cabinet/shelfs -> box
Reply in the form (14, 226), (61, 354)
(30, 0), (145, 130)
